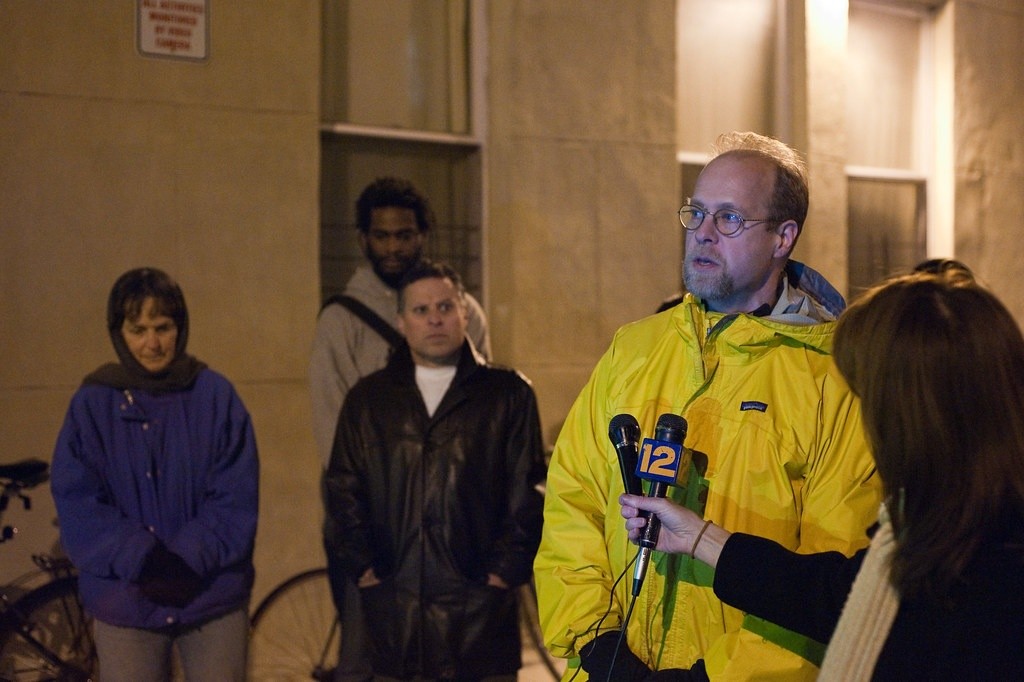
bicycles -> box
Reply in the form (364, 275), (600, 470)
(245, 565), (344, 682)
(0, 457), (96, 682)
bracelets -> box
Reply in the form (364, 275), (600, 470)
(692, 520), (714, 553)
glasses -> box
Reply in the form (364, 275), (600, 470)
(677, 205), (786, 235)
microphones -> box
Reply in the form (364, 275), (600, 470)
(632, 413), (688, 597)
(608, 414), (648, 547)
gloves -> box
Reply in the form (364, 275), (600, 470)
(140, 541), (206, 606)
(577, 630), (710, 682)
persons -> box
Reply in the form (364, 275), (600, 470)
(327, 259), (547, 682)
(531, 134), (886, 682)
(312, 177), (493, 472)
(619, 276), (1024, 682)
(50, 267), (261, 682)
